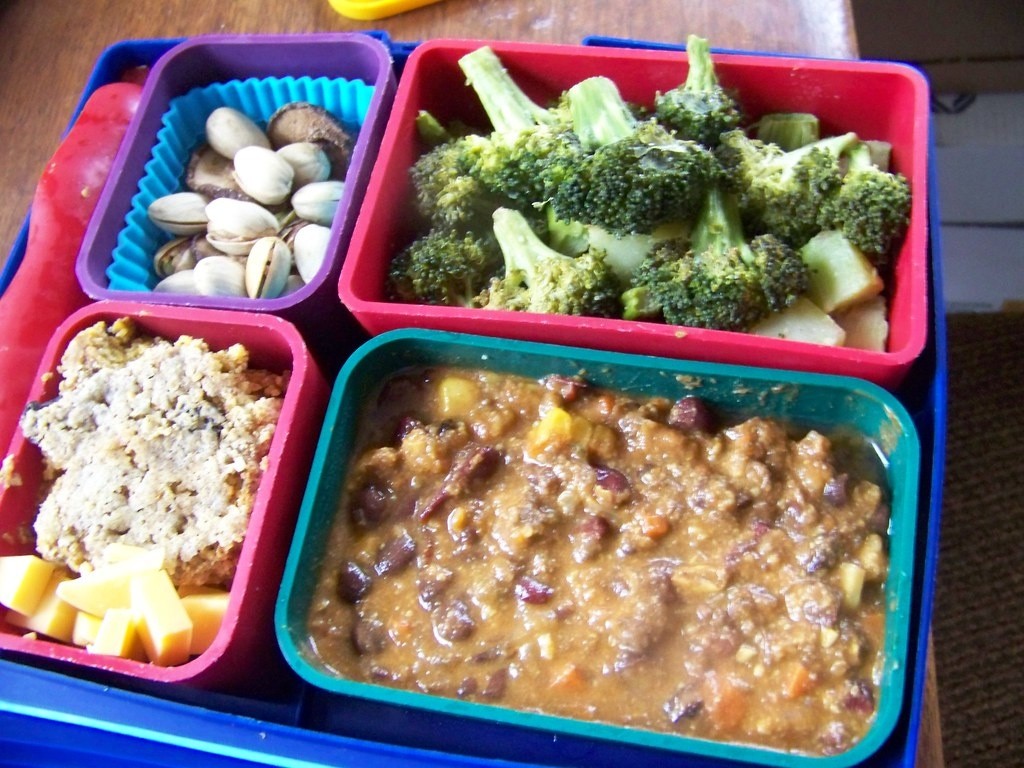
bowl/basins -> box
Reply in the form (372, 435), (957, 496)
(0, 301), (332, 691)
(274, 328), (920, 768)
(76, 34), (400, 326)
(338, 35), (929, 387)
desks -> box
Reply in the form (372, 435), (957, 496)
(0, 1), (944, 767)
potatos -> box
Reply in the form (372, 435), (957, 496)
(0, 554), (231, 668)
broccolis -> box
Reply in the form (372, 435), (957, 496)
(375, 35), (912, 352)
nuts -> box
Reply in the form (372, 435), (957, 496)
(147, 107), (346, 301)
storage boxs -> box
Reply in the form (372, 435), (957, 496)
(0, 28), (949, 767)
(275, 326), (922, 768)
(345, 38), (931, 384)
(75, 33), (397, 354)
(1, 302), (331, 701)
